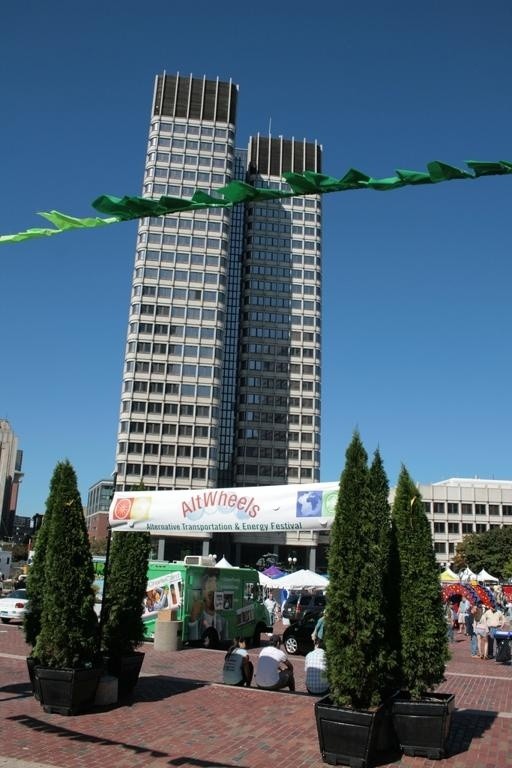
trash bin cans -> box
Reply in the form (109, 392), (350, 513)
(153, 620), (183, 652)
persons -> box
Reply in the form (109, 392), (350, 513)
(303, 638), (332, 695)
(265, 589), (278, 626)
(188, 567), (229, 640)
(442, 595), (512, 659)
(310, 609), (329, 640)
(255, 633), (296, 691)
(223, 635), (254, 688)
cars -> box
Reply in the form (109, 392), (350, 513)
(0, 572), (33, 624)
(281, 594), (326, 654)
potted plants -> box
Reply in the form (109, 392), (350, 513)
(28, 458), (150, 716)
(388, 462), (455, 762)
(314, 432), (393, 763)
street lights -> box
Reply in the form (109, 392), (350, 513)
(288, 551), (298, 574)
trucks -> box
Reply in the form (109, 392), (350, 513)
(92, 555), (273, 648)
